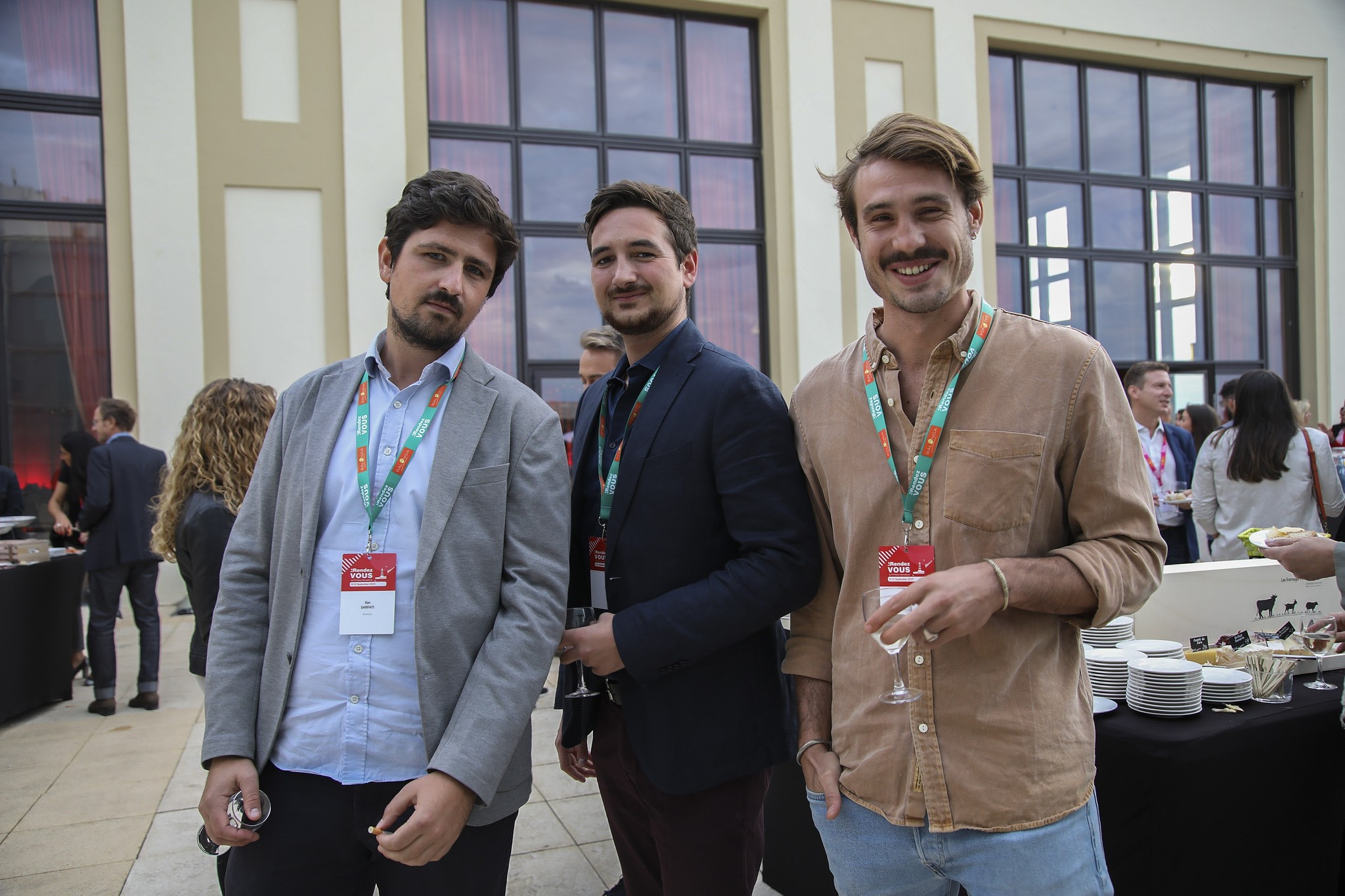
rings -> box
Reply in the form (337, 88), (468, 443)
(921, 624), (939, 642)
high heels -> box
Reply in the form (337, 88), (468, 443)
(73, 656), (88, 678)
(83, 673), (94, 686)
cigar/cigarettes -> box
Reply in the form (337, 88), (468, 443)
(368, 826), (394, 838)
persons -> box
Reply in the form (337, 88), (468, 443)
(1122, 361), (1199, 565)
(1293, 400), (1311, 427)
(0, 464), (25, 541)
(575, 325), (626, 390)
(785, 111), (1168, 896)
(1175, 402), (1223, 457)
(147, 376), (278, 698)
(1259, 535), (1345, 654)
(1174, 408), (1187, 426)
(1317, 399), (1345, 447)
(190, 168), (575, 896)
(1218, 378), (1239, 421)
(47, 429), (98, 686)
(75, 397), (169, 718)
(548, 178), (826, 896)
(1188, 368), (1345, 563)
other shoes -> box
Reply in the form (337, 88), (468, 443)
(88, 698), (116, 715)
(128, 692), (159, 710)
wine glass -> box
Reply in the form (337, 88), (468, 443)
(197, 789), (271, 856)
(1171, 480), (1188, 514)
(562, 607), (602, 698)
(863, 586), (924, 704)
(1299, 614), (1338, 690)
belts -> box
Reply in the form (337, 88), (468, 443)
(601, 678), (621, 707)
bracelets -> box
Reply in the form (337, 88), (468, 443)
(795, 738), (832, 766)
(982, 557), (1010, 611)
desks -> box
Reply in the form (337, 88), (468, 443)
(0, 547), (89, 725)
(1089, 645), (1344, 896)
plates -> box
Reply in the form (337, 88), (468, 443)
(1075, 615), (1252, 716)
(1249, 529), (1324, 548)
(0, 516), (36, 528)
(1158, 497), (1193, 503)
(0, 523), (15, 534)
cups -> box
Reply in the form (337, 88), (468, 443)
(1245, 662), (1293, 703)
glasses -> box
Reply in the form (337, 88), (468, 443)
(1219, 399), (1230, 407)
(92, 418), (102, 424)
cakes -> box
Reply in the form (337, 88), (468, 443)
(1265, 525), (1319, 541)
(1184, 630), (1310, 668)
(1167, 492), (1187, 500)
